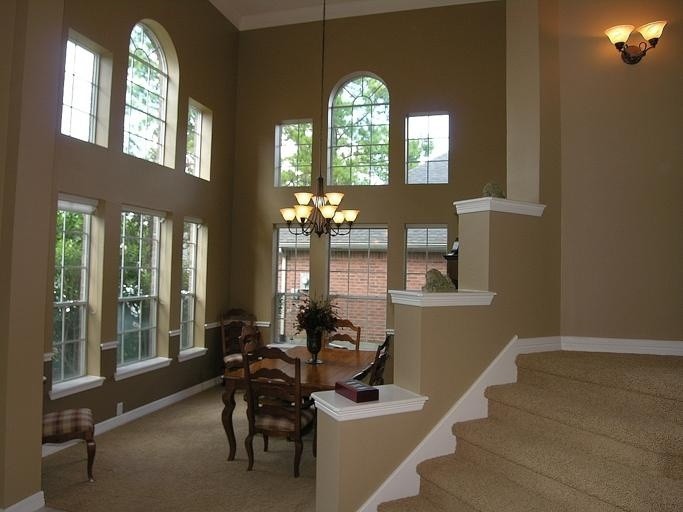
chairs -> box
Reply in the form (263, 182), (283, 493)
(42, 407), (96, 483)
(221, 308), (391, 478)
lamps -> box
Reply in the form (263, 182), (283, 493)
(604, 21), (667, 65)
(279, 0), (361, 239)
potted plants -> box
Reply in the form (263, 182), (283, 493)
(291, 293), (343, 365)
